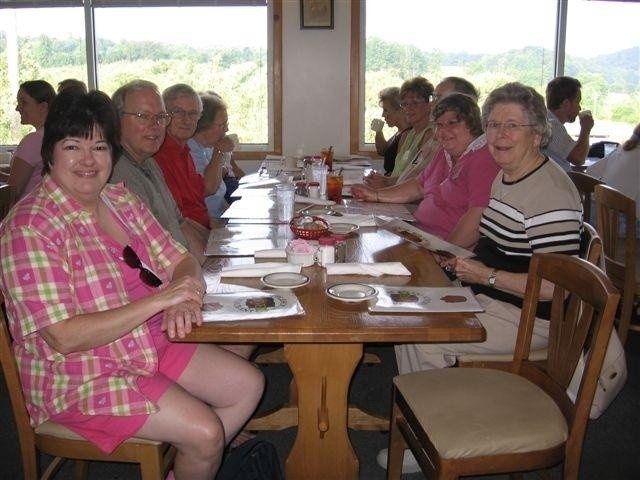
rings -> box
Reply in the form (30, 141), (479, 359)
(196, 287), (201, 294)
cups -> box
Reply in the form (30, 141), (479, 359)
(328, 176), (343, 204)
(371, 119), (385, 131)
(578, 110), (591, 119)
(223, 134), (240, 152)
(320, 148), (334, 172)
(276, 187), (295, 222)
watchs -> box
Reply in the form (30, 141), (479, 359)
(488, 270), (498, 288)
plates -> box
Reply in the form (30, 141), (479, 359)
(328, 223), (360, 234)
(325, 282), (379, 302)
(260, 272), (310, 288)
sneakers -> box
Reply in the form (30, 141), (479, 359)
(374, 449), (428, 474)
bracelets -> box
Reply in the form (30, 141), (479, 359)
(376, 192), (379, 204)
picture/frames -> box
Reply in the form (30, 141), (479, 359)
(300, 1), (334, 28)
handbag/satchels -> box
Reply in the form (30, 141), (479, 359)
(567, 327), (628, 420)
(216, 438), (284, 480)
(588, 141), (619, 159)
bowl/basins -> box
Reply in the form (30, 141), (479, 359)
(286, 251), (314, 268)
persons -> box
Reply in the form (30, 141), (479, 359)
(1, 86), (266, 479)
(56, 80), (87, 92)
(110, 79), (206, 277)
(363, 79), (480, 186)
(351, 93), (500, 274)
(587, 125), (639, 238)
(9, 82), (56, 204)
(155, 83), (244, 230)
(545, 76), (593, 173)
(376, 83), (585, 474)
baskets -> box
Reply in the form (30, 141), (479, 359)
(290, 215), (329, 239)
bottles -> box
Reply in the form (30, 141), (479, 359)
(296, 182), (307, 196)
(318, 238), (334, 267)
(330, 234), (347, 263)
(307, 182), (320, 198)
(303, 155), (322, 167)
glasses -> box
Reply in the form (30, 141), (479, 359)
(486, 121), (535, 130)
(171, 107), (201, 119)
(122, 245), (162, 287)
(123, 111), (171, 127)
